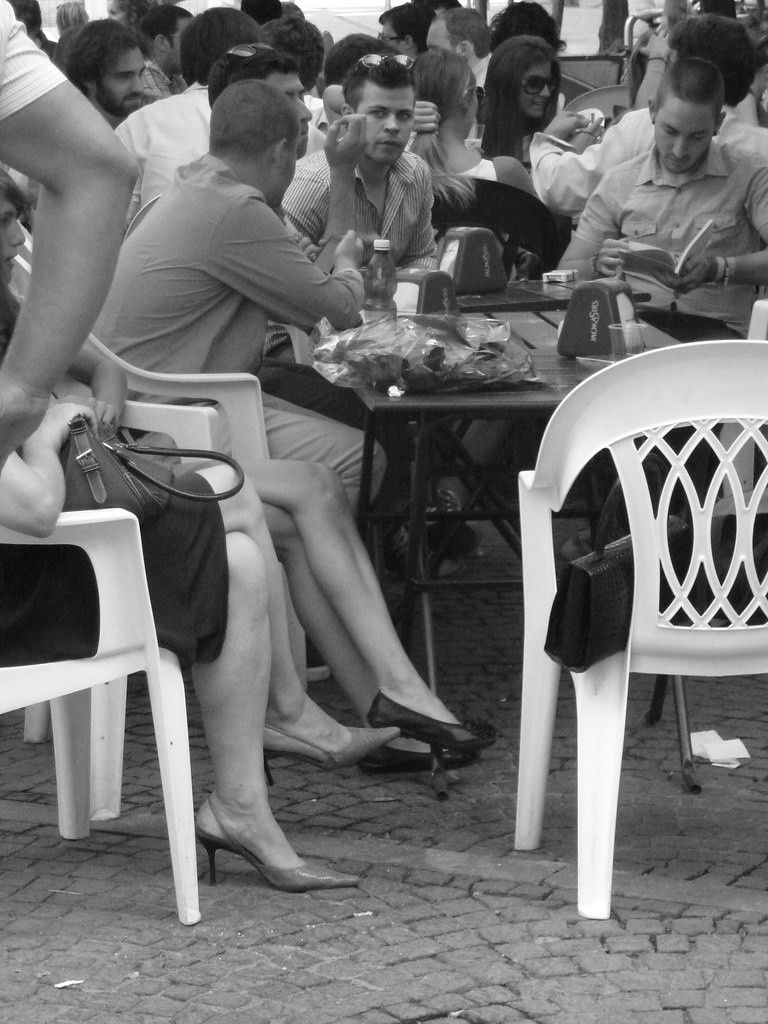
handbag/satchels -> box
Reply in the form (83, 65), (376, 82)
(47, 415), (244, 667)
(546, 450), (691, 674)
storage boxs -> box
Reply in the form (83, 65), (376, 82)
(542, 270), (579, 283)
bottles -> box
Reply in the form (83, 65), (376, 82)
(363, 239), (398, 324)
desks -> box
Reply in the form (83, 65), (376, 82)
(342, 271), (687, 803)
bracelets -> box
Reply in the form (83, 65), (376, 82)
(646, 57), (668, 64)
(726, 256), (736, 279)
(520, 251), (540, 262)
(715, 256), (725, 280)
(723, 259), (730, 285)
(572, 129), (597, 138)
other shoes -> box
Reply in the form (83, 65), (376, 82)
(360, 736), (480, 771)
(303, 635), (330, 681)
(561, 513), (634, 560)
(385, 542), (461, 579)
(400, 517), (483, 553)
(368, 692), (498, 751)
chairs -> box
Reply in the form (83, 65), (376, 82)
(515, 299), (768, 921)
(0, 217), (307, 926)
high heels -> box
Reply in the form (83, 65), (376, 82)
(262, 724), (401, 786)
(194, 797), (358, 891)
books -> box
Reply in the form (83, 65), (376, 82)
(618, 219), (714, 299)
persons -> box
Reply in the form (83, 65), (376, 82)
(0, 0), (768, 892)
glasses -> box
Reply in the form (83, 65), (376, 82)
(664, 52), (673, 71)
(378, 33), (403, 44)
(518, 74), (558, 95)
(469, 86), (486, 106)
(223, 42), (274, 86)
(344, 55), (416, 94)
